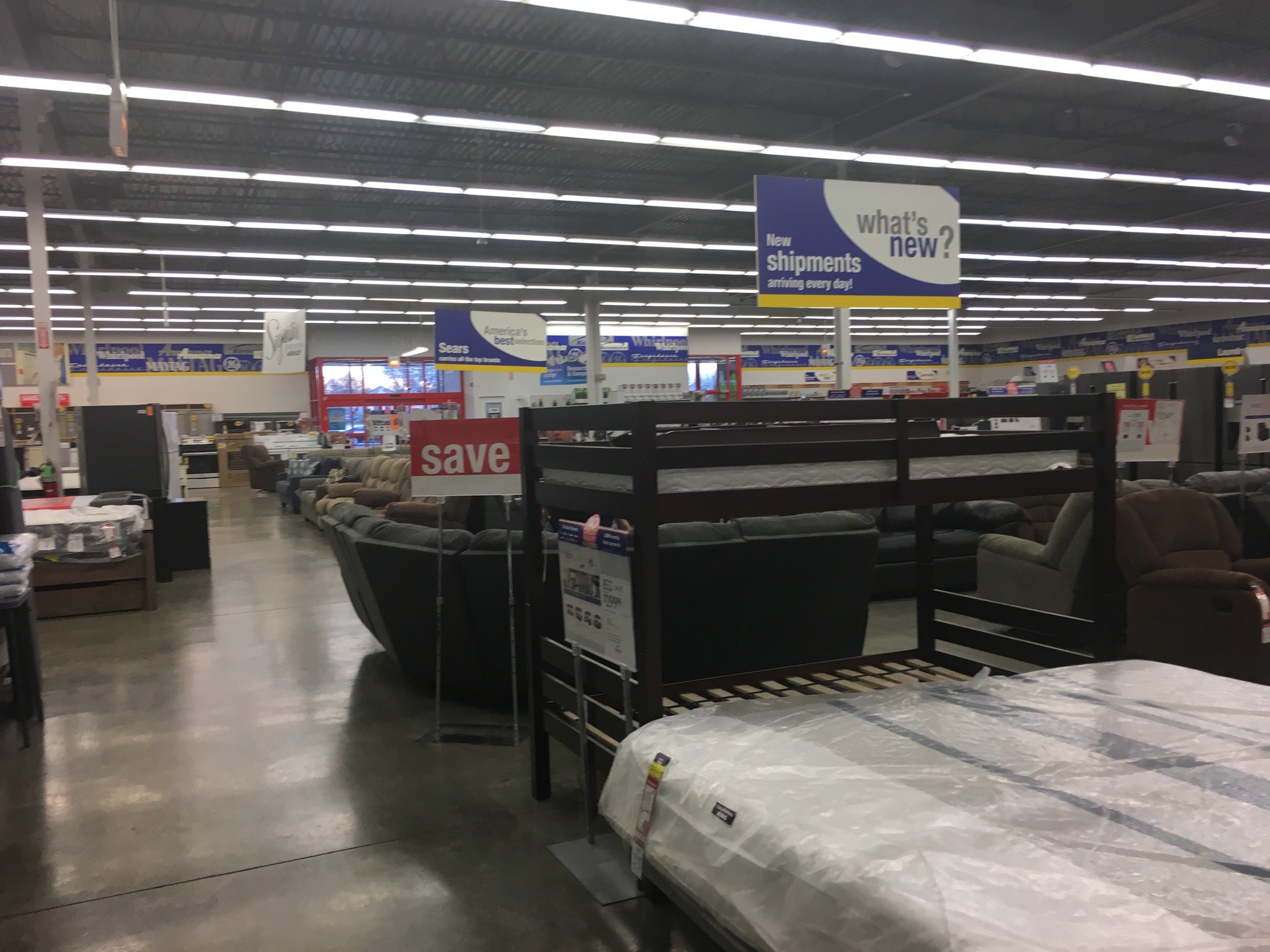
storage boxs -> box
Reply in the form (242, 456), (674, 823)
(215, 433), (253, 488)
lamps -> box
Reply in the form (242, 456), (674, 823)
(0, 0), (1270, 334)
(400, 346), (429, 356)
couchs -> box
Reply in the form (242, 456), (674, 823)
(245, 445), (1270, 715)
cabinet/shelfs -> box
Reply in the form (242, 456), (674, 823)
(30, 533), (156, 625)
(165, 496), (212, 571)
(0, 389), (44, 749)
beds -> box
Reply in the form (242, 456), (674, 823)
(518, 393), (1270, 952)
